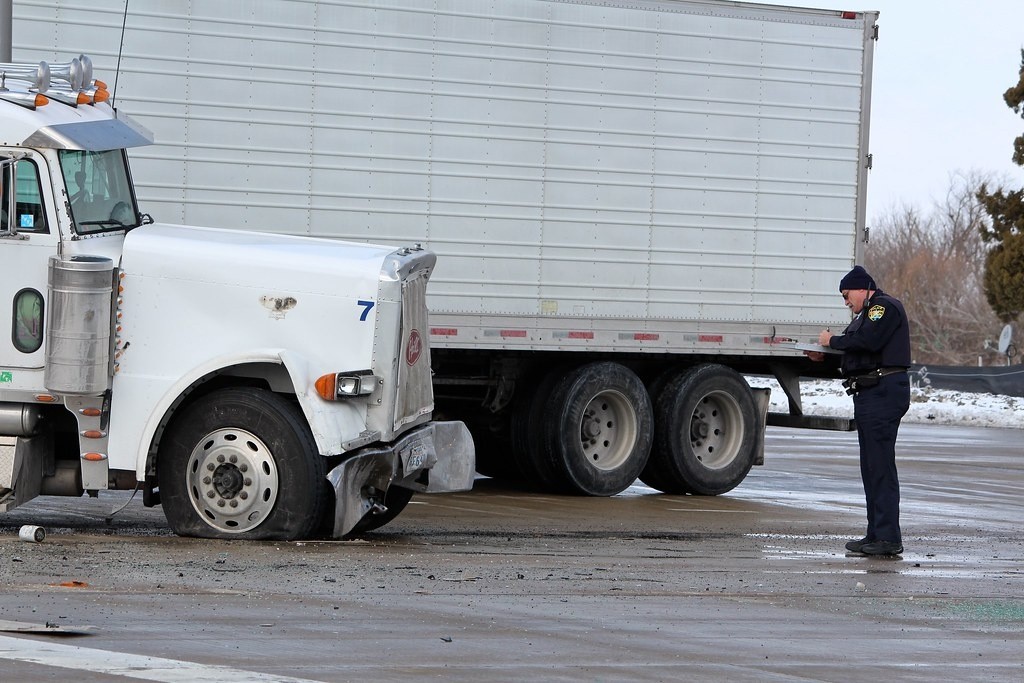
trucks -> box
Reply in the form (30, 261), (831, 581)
(0, 56), (493, 540)
(0, 0), (881, 496)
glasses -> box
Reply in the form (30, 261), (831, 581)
(843, 290), (850, 298)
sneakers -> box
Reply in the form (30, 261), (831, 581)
(860, 540), (904, 555)
(845, 538), (876, 552)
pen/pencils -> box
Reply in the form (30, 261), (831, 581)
(827, 327), (829, 332)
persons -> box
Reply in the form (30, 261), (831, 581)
(802, 266), (911, 554)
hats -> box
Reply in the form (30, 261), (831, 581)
(839, 265), (876, 293)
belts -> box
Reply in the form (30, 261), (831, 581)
(868, 366), (907, 377)
